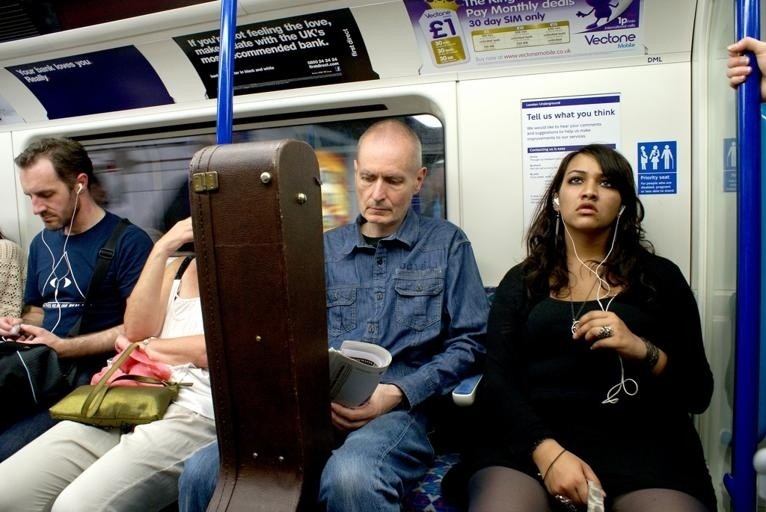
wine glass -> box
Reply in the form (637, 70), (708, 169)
(553, 493), (574, 509)
(593, 325), (611, 343)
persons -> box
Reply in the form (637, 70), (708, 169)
(0, 215), (218, 512)
(0, 228), (24, 340)
(175, 115), (493, 510)
(0, 134), (157, 464)
(723, 35), (765, 102)
(437, 140), (721, 512)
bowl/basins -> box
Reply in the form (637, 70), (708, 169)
(564, 274), (605, 338)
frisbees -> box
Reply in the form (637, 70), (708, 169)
(77, 183), (83, 195)
(618, 205), (626, 216)
(554, 197), (560, 206)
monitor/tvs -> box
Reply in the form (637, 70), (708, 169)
(327, 337), (395, 407)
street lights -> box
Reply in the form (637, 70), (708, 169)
(0, 340), (64, 434)
(48, 342), (201, 428)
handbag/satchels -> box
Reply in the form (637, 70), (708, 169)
(542, 449), (569, 486)
(139, 336), (158, 352)
(636, 333), (661, 374)
